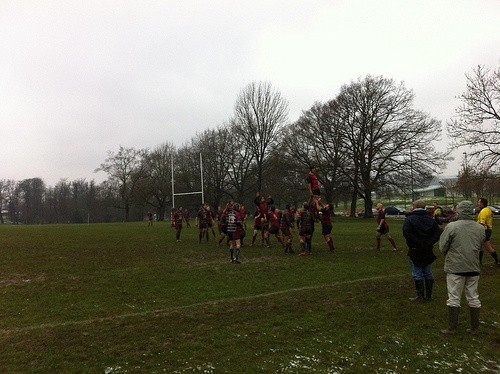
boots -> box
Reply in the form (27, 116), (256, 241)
(441, 306), (461, 335)
(465, 307), (480, 335)
(408, 280), (433, 304)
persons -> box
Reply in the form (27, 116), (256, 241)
(433, 202), (443, 229)
(403, 200), (440, 301)
(476, 198), (500, 265)
(375, 203), (399, 252)
(170, 166), (334, 264)
(438, 200), (485, 335)
(146, 210), (153, 228)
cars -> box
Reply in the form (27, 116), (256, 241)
(385, 205), (408, 214)
(426, 203), (500, 217)
(334, 208), (377, 216)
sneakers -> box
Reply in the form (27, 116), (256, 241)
(231, 258), (241, 264)
(490, 263), (500, 268)
(299, 252), (306, 257)
(392, 247), (399, 252)
